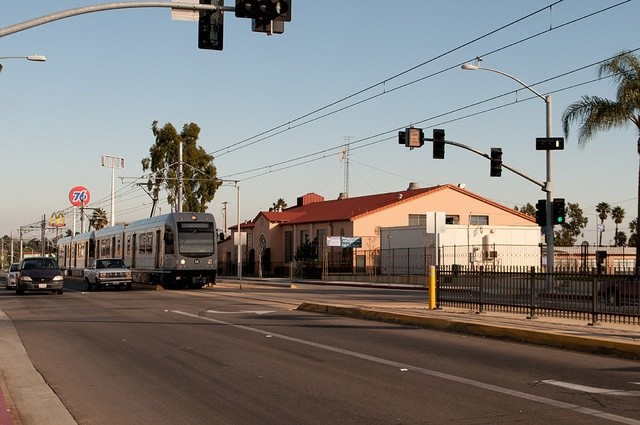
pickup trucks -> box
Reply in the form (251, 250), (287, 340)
(84, 258), (132, 288)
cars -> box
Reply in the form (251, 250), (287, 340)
(5, 262), (19, 288)
(16, 256), (66, 294)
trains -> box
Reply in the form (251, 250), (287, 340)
(57, 212), (224, 288)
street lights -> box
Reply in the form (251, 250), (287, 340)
(461, 56), (554, 292)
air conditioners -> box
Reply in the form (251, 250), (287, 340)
(485, 251), (498, 260)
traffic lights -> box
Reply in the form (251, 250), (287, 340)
(536, 199), (546, 226)
(553, 198), (566, 224)
(235, 0), (291, 20)
(491, 148), (502, 176)
(433, 128), (444, 158)
(536, 137), (564, 150)
